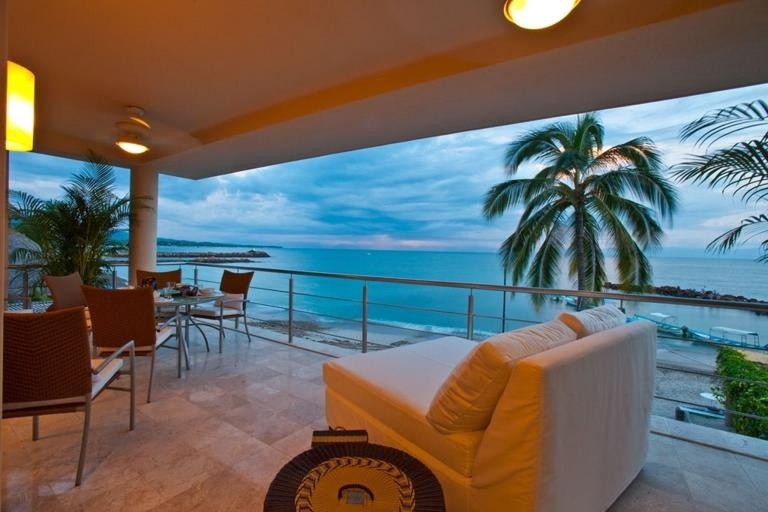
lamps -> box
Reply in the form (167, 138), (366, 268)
(6, 59), (36, 152)
(112, 105), (153, 155)
(498, 0), (586, 32)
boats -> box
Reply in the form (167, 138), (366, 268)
(547, 288), (767, 354)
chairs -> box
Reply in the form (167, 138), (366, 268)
(3, 307), (135, 486)
(43, 271), (84, 312)
(322, 305), (656, 512)
(137, 268), (181, 288)
(191, 270), (254, 353)
(81, 283), (181, 403)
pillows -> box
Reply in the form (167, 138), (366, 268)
(425, 304), (626, 435)
